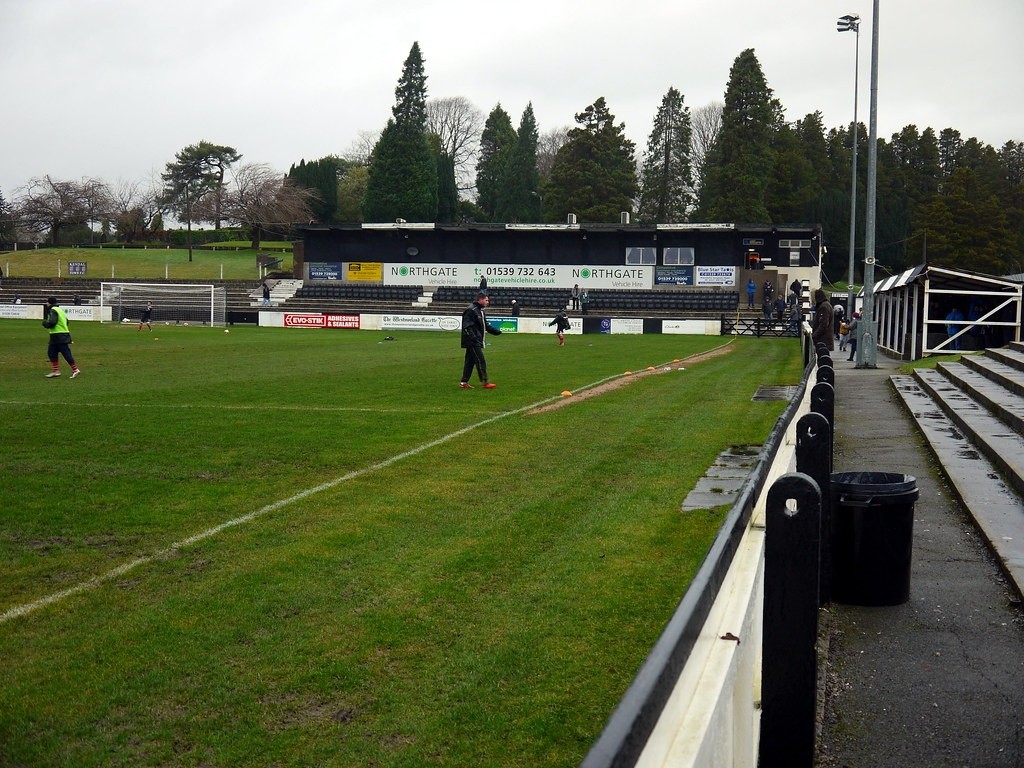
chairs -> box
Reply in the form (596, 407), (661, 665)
(296, 282), (741, 312)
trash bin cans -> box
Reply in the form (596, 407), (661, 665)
(74, 295), (81, 306)
(823, 472), (920, 607)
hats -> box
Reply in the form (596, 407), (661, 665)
(852, 313), (859, 318)
(48, 298), (56, 305)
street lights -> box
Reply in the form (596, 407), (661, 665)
(836, 12), (862, 322)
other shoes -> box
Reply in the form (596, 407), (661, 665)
(844, 348), (846, 351)
(840, 347), (842, 350)
(847, 359), (852, 361)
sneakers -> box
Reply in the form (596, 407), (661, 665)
(459, 383), (475, 389)
(45, 372), (61, 378)
(69, 369), (80, 379)
(482, 383), (496, 389)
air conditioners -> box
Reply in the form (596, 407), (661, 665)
(621, 212), (630, 224)
(567, 213), (576, 224)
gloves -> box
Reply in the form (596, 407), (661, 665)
(497, 331), (501, 335)
(472, 337), (479, 343)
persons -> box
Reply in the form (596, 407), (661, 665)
(788, 293), (802, 331)
(747, 279), (756, 307)
(835, 308), (850, 351)
(762, 295), (787, 319)
(842, 312), (860, 361)
(790, 279), (801, 297)
(811, 288), (834, 351)
(0, 267), (3, 279)
(42, 297), (80, 379)
(511, 299), (519, 317)
(945, 306), (964, 350)
(137, 301), (153, 333)
(13, 291), (21, 303)
(763, 279), (774, 295)
(72, 294), (81, 306)
(458, 275), (501, 389)
(260, 283), (272, 306)
(571, 284), (589, 315)
(549, 308), (570, 346)
(969, 296), (987, 334)
